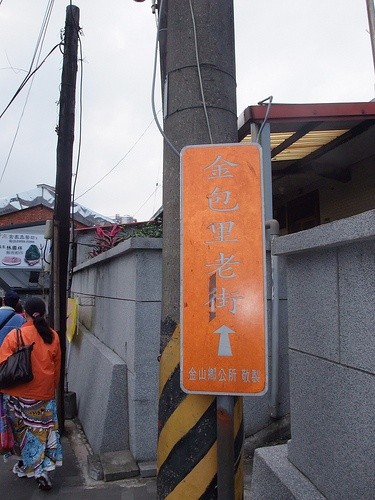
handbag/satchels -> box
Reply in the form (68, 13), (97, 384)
(0, 346), (33, 388)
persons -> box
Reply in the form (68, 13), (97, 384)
(0, 294), (27, 320)
(0, 290), (27, 349)
(0, 297), (64, 493)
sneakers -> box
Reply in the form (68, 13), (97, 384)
(35, 470), (51, 489)
(12, 460), (26, 477)
